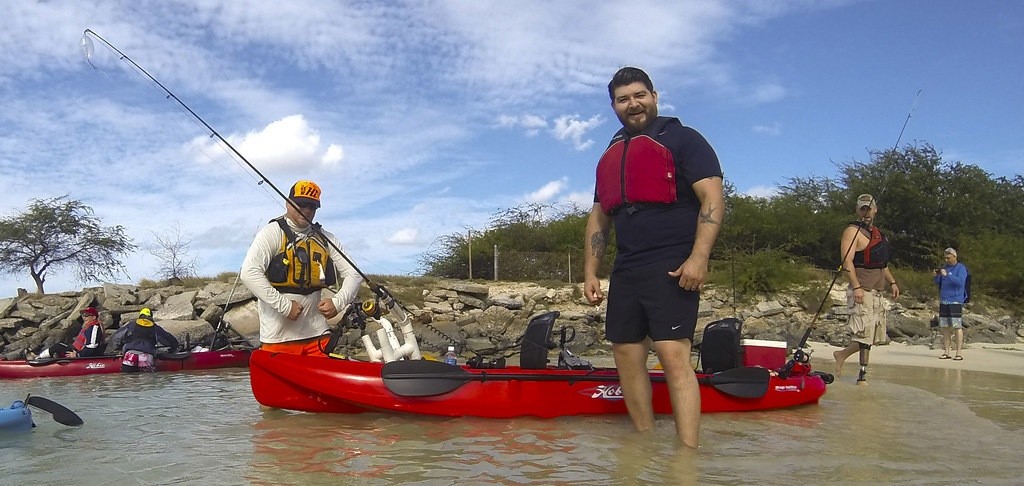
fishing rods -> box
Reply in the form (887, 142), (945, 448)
(775, 86), (923, 379)
(208, 215), (265, 353)
(79, 26), (399, 322)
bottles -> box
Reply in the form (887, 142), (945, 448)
(445, 346), (457, 366)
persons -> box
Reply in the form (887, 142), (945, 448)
(24, 307), (106, 361)
(239, 180), (362, 357)
(933, 248), (967, 360)
(583, 67), (726, 448)
(834, 194), (899, 383)
(105, 308), (178, 373)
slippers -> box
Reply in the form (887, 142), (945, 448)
(939, 354), (951, 359)
(953, 356), (963, 360)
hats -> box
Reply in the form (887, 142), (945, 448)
(289, 179), (322, 208)
(857, 194), (875, 210)
(82, 307), (98, 318)
(140, 308), (153, 317)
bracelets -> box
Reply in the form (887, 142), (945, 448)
(890, 283), (896, 286)
(853, 287), (861, 290)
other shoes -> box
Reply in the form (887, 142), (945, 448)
(25, 348), (37, 360)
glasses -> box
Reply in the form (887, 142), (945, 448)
(82, 314), (94, 317)
(297, 203), (318, 210)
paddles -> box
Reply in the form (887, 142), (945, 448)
(28, 351), (192, 367)
(154, 339), (246, 348)
(380, 358), (772, 401)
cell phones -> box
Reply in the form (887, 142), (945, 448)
(936, 268), (941, 275)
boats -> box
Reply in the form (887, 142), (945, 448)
(249, 348), (834, 420)
(0, 350), (250, 380)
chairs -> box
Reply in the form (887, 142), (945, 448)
(519, 310), (560, 369)
(700, 317), (743, 374)
(85, 340), (109, 356)
